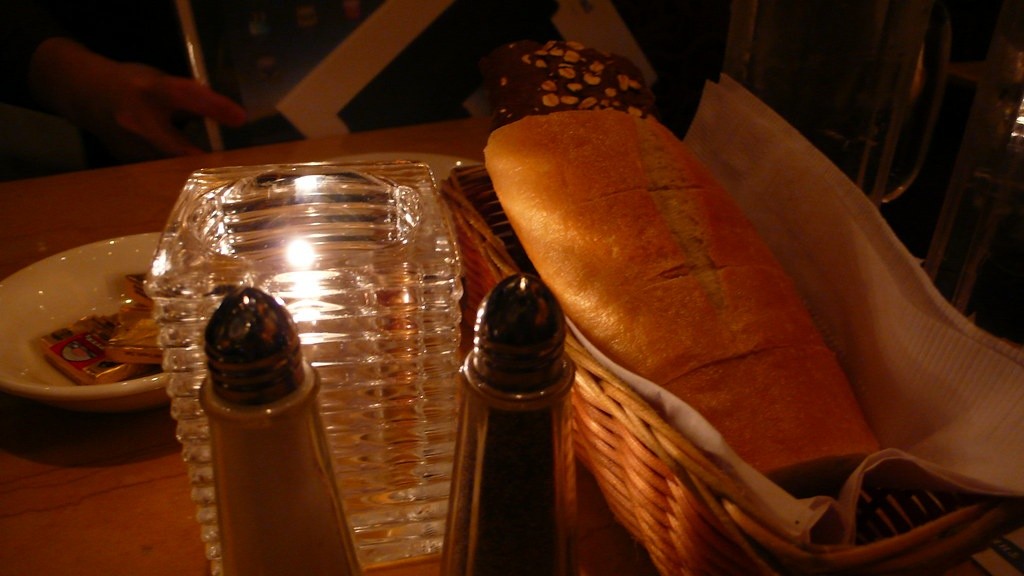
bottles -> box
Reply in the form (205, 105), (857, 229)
(199, 287), (364, 576)
(440, 271), (579, 576)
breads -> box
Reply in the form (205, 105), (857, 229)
(483, 112), (880, 480)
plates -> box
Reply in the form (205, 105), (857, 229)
(0, 231), (167, 412)
(331, 150), (485, 191)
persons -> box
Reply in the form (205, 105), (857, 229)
(0, 0), (566, 161)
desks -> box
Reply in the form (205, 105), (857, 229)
(0, 114), (654, 576)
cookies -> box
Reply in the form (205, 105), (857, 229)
(477, 39), (650, 128)
